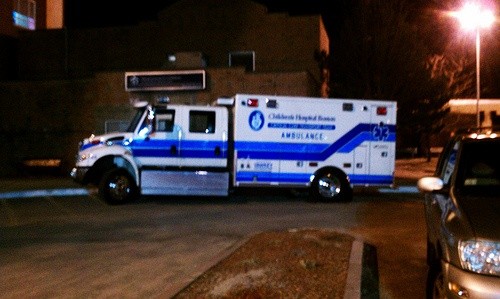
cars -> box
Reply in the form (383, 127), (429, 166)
(417, 135), (500, 298)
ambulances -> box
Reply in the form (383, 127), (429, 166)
(69, 94), (398, 205)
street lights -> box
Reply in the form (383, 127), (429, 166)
(459, 3), (494, 131)
